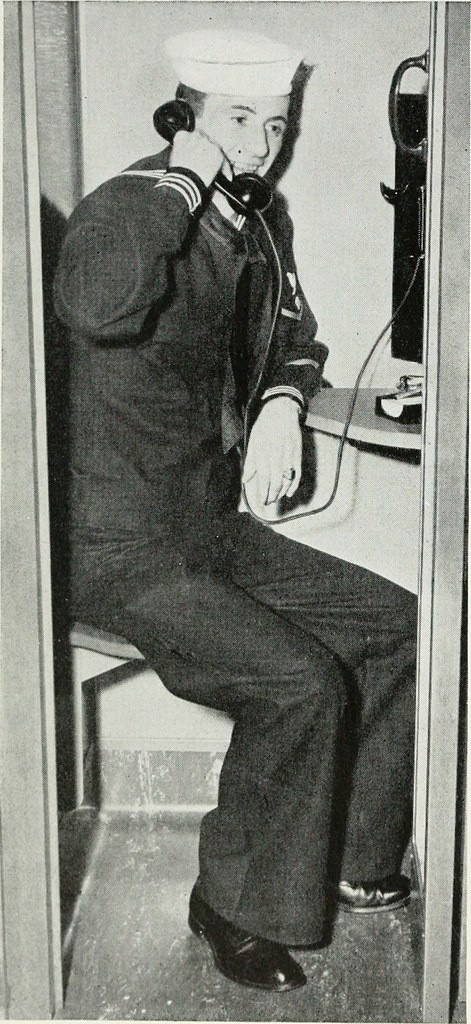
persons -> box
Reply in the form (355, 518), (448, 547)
(56, 28), (418, 989)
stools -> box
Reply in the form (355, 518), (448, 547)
(56, 620), (147, 808)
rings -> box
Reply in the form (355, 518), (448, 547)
(283, 468), (296, 481)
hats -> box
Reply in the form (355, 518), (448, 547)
(162, 29), (304, 98)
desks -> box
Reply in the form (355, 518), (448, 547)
(302, 387), (422, 452)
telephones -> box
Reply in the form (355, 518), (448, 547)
(151, 96), (271, 215)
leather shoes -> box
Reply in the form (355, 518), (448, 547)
(327, 874), (412, 915)
(188, 886), (306, 993)
